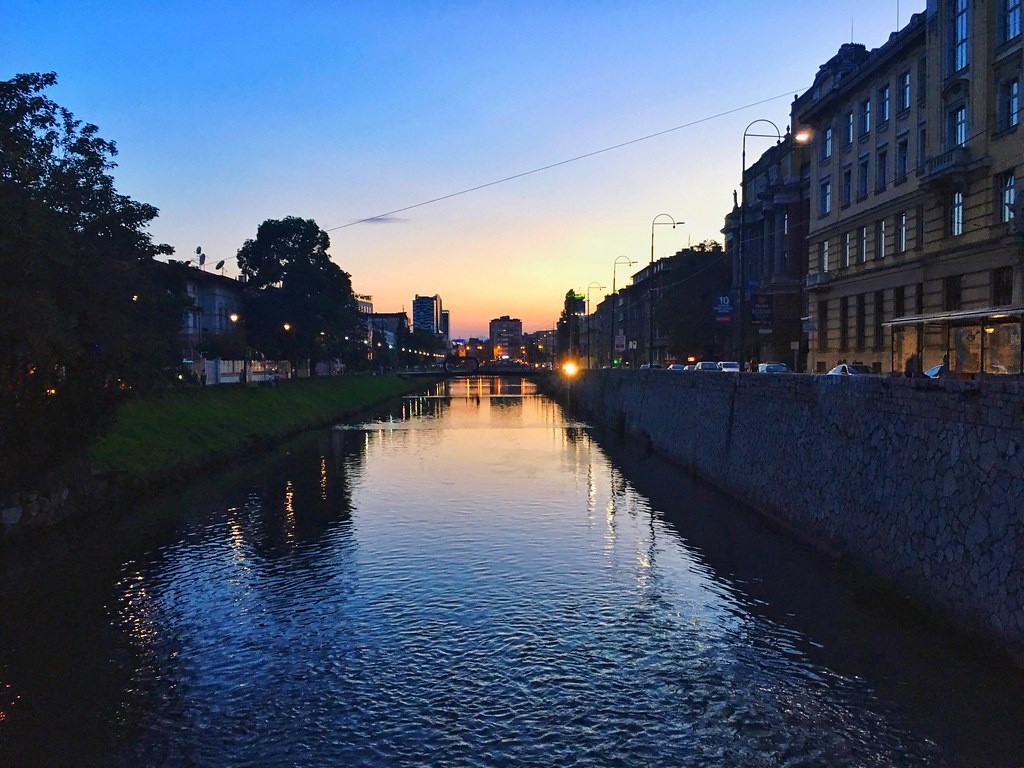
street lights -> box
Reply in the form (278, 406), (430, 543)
(610, 255), (638, 367)
(649, 213), (690, 365)
(738, 119), (810, 372)
(588, 282), (607, 369)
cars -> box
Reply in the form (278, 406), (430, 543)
(641, 361), (739, 371)
(756, 362), (793, 373)
(827, 363), (884, 376)
